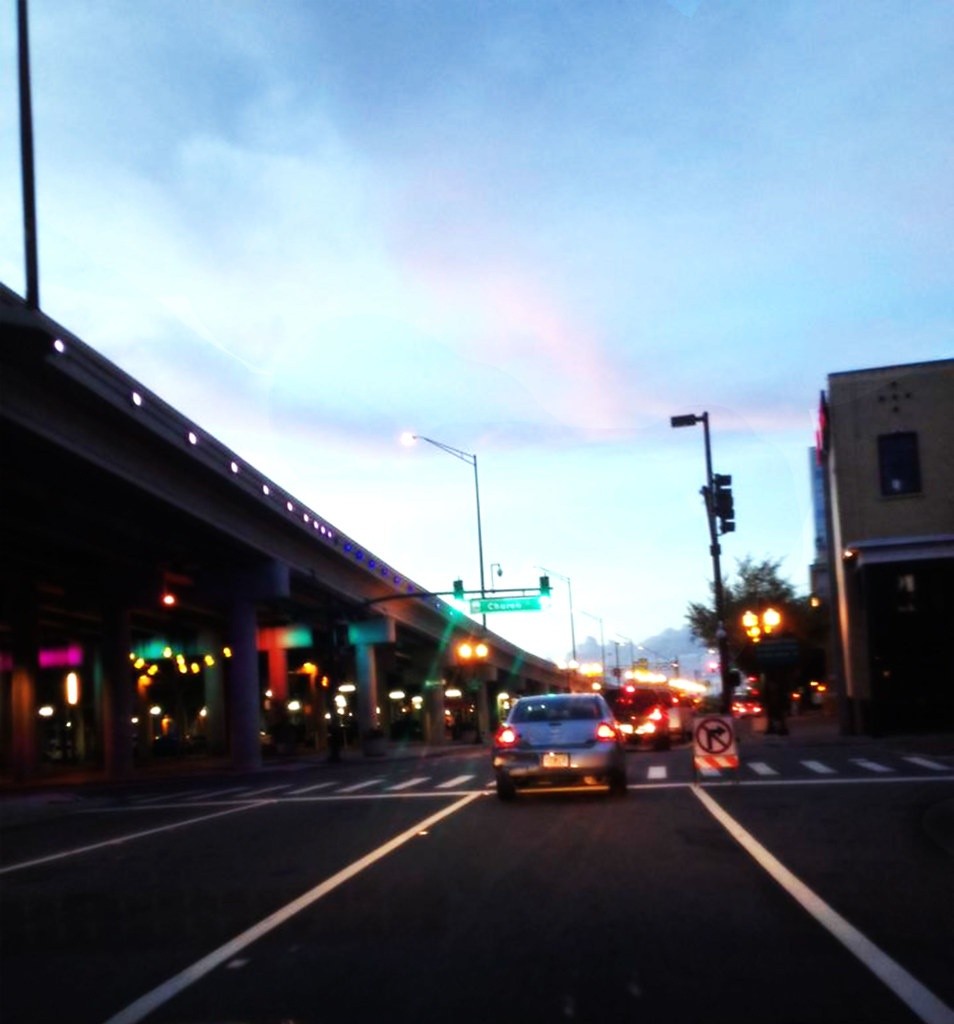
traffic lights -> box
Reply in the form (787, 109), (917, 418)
(453, 639), (497, 665)
(711, 471), (736, 533)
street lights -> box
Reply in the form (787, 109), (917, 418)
(670, 411), (735, 717)
(489, 561), (505, 590)
(533, 566), (576, 668)
(403, 431), (488, 634)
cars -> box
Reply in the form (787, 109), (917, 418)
(490, 688), (625, 801)
(657, 689), (695, 742)
(607, 687), (675, 753)
